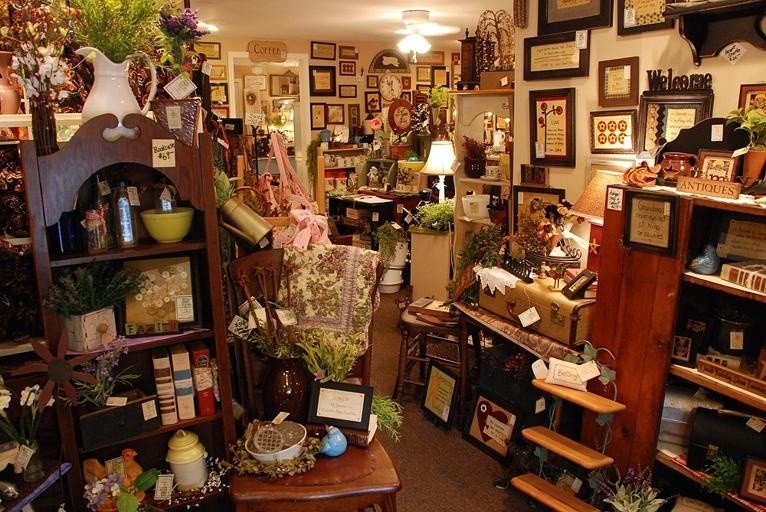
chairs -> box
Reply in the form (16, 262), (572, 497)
(229, 249), (403, 512)
(391, 247), (485, 432)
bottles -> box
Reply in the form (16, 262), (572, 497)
(154, 178), (177, 213)
(655, 151), (700, 187)
(0, 51), (20, 116)
(164, 429), (209, 492)
(84, 170), (138, 256)
(320, 126), (330, 142)
(324, 171), (348, 191)
(362, 111), (375, 143)
(486, 155), (500, 179)
(0, 151), (40, 343)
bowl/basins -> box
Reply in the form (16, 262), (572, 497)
(246, 419), (308, 466)
(141, 207), (191, 244)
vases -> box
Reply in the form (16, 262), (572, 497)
(376, 220), (409, 269)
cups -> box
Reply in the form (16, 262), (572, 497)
(492, 130), (505, 146)
(219, 198), (274, 248)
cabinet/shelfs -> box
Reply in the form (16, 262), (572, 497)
(447, 87), (515, 279)
(329, 186), (394, 252)
(408, 224), (454, 303)
(578, 186), (766, 512)
(312, 138), (370, 215)
(358, 184), (429, 233)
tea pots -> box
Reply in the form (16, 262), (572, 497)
(74, 45), (159, 139)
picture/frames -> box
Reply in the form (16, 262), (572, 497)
(513, 185), (566, 239)
(617, 0), (676, 36)
(636, 68), (715, 160)
(623, 191), (679, 256)
(529, 86), (576, 168)
(261, 100), (271, 118)
(523, 29), (591, 82)
(589, 109), (637, 153)
(695, 147), (740, 183)
(420, 358), (462, 432)
(308, 40), (460, 138)
(537, 0), (614, 36)
(584, 160), (635, 189)
(738, 82), (766, 121)
(242, 74), (268, 93)
(305, 380), (374, 434)
(598, 55), (640, 108)
(269, 74), (301, 98)
(460, 386), (522, 465)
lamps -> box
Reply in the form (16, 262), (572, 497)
(395, 10), (432, 63)
(420, 140), (456, 203)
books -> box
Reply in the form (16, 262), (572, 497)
(307, 382), (378, 432)
(169, 344), (196, 419)
(719, 257), (766, 293)
(303, 411), (379, 447)
(150, 347), (178, 426)
(187, 342), (216, 418)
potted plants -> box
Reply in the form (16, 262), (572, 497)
(397, 149), (429, 192)
(723, 107), (766, 187)
(463, 135), (494, 179)
(247, 304), (310, 426)
(211, 141), (273, 250)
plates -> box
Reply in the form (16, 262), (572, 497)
(479, 175), (500, 181)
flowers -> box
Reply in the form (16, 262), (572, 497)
(589, 464), (680, 512)
